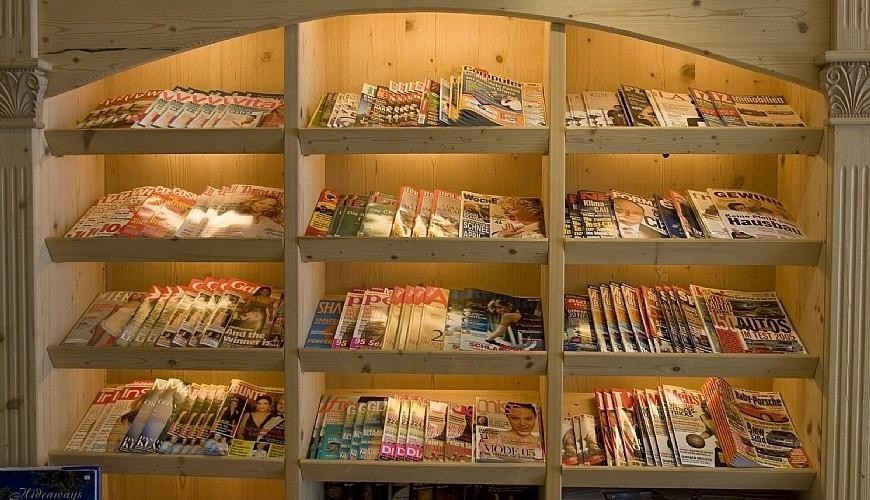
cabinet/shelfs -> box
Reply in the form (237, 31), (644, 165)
(1, 1), (869, 498)
